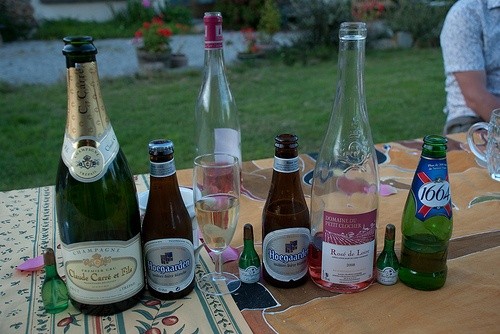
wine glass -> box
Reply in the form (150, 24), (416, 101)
(192, 153), (241, 295)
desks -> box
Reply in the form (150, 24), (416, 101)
(0, 132), (500, 334)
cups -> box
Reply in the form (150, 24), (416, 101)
(466, 108), (500, 182)
(138, 187), (205, 265)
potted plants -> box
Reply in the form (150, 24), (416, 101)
(259, 1), (283, 60)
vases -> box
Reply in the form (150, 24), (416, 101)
(136, 47), (171, 77)
(239, 51), (263, 68)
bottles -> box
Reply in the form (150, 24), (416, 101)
(196, 12), (241, 181)
(398, 135), (454, 291)
(55, 36), (145, 316)
(308, 22), (378, 293)
(42, 248), (68, 313)
(376, 224), (399, 286)
(141, 138), (194, 299)
(238, 224), (261, 285)
(262, 133), (310, 288)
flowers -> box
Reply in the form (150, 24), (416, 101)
(132, 0), (175, 50)
(228, 27), (260, 52)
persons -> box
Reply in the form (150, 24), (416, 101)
(440, 0), (500, 135)
